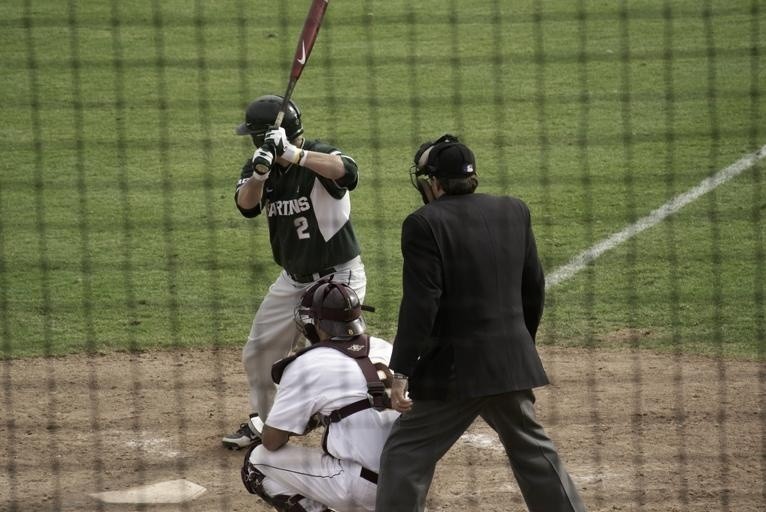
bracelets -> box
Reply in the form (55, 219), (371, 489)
(392, 374), (409, 380)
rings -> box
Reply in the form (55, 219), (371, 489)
(397, 400), (400, 404)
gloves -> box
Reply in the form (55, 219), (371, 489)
(251, 143), (274, 181)
(263, 126), (305, 166)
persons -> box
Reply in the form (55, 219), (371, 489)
(375, 131), (589, 510)
(239, 278), (409, 511)
(219, 93), (372, 459)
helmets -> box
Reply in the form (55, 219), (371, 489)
(409, 131), (478, 205)
(292, 269), (368, 340)
(234, 94), (304, 142)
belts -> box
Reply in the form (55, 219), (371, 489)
(287, 266), (336, 283)
(337, 459), (378, 485)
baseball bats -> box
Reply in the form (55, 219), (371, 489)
(256, 0), (329, 174)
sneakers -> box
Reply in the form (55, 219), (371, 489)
(220, 422), (263, 451)
